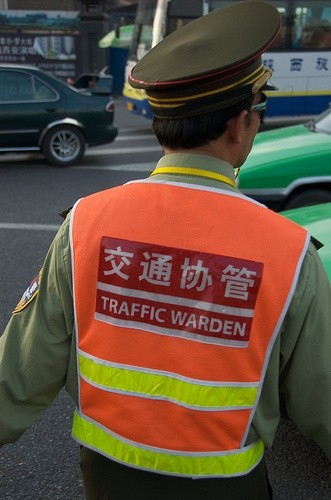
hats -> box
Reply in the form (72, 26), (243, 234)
(128, 1), (282, 120)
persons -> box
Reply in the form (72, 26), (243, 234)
(270, 17), (331, 50)
(0, 1), (331, 500)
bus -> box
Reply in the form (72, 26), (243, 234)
(123, 0), (331, 129)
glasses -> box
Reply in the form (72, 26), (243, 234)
(250, 93), (268, 119)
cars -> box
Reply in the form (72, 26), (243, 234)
(235, 106), (330, 283)
(0, 64), (118, 166)
